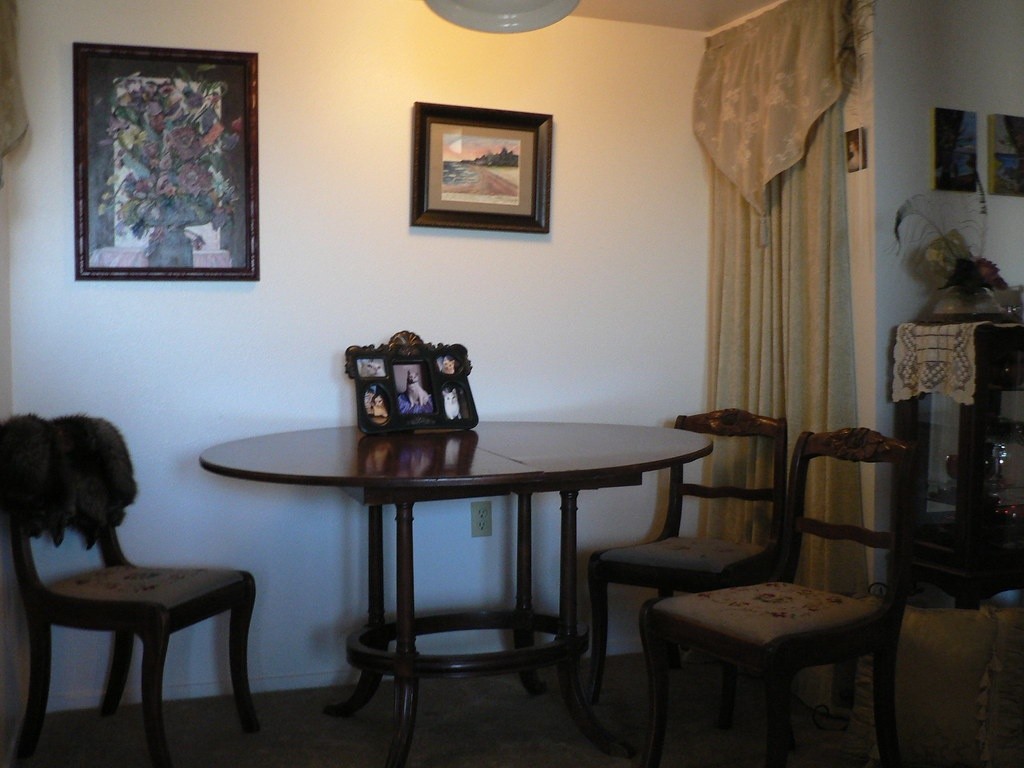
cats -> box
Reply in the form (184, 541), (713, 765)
(442, 387), (463, 420)
(359, 357), (387, 378)
(441, 357), (456, 373)
(367, 388), (388, 419)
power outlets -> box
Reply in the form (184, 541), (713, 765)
(471, 501), (492, 536)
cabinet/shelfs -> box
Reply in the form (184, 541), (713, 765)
(893, 323), (1024, 609)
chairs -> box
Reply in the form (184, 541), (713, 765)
(589, 409), (913, 768)
(0, 414), (259, 768)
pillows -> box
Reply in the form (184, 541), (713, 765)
(866, 605), (1024, 768)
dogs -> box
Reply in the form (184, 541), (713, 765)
(406, 369), (429, 407)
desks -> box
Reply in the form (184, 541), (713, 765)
(201, 421), (714, 768)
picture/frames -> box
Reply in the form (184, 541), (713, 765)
(411, 102), (553, 233)
(73, 42), (260, 280)
(345, 331), (478, 436)
